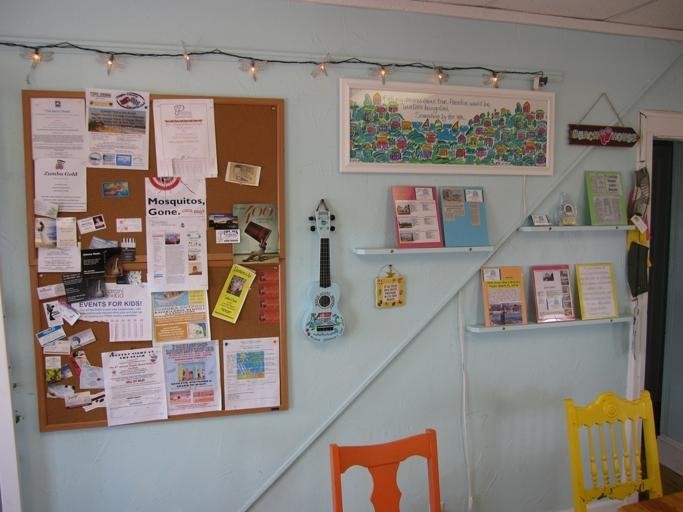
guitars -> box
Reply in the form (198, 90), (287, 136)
(301, 199), (344, 342)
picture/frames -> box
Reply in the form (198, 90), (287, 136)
(336, 75), (556, 175)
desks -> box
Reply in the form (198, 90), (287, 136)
(616, 489), (682, 512)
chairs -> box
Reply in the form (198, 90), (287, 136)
(329, 428), (441, 512)
(564, 390), (661, 512)
